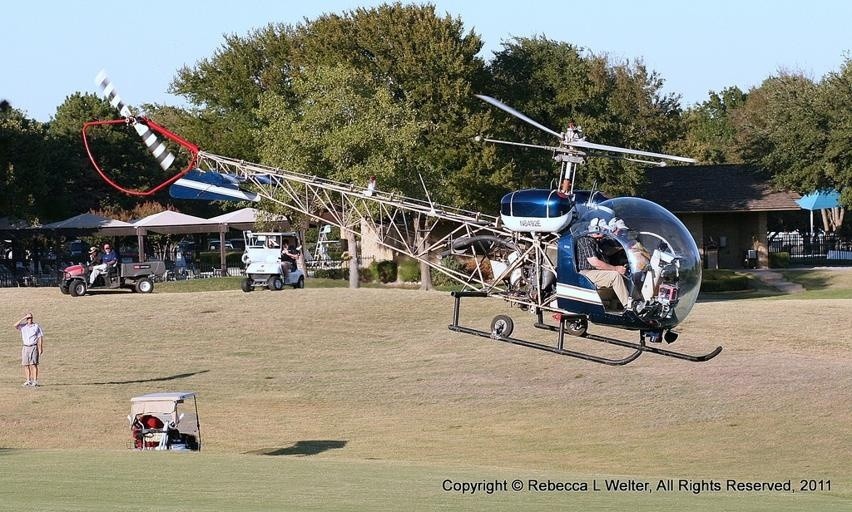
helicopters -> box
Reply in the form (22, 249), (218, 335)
(81, 68), (722, 366)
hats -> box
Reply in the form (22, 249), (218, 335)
(589, 217), (609, 230)
(608, 216), (629, 231)
(87, 246), (99, 253)
(103, 244), (109, 249)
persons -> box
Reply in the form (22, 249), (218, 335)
(577, 218), (642, 309)
(15, 313), (43, 388)
(282, 239), (299, 283)
(87, 244), (118, 288)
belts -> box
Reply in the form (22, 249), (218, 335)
(23, 343), (37, 347)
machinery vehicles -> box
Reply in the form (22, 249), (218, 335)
(59, 256), (166, 297)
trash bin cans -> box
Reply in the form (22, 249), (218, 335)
(707, 249), (718, 269)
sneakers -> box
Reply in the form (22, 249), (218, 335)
(634, 300), (657, 316)
(20, 380), (37, 386)
